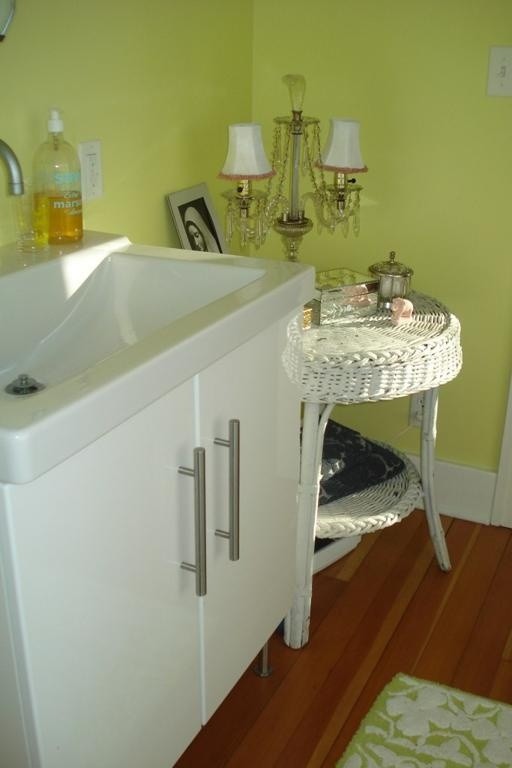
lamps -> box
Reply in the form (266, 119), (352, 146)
(218, 73), (368, 261)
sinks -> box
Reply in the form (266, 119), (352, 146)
(0, 227), (316, 460)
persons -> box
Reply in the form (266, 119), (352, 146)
(186, 220), (208, 252)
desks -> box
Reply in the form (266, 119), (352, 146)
(282, 288), (462, 645)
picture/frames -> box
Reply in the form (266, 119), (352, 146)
(166, 182), (229, 255)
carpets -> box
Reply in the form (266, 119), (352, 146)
(334, 670), (512, 766)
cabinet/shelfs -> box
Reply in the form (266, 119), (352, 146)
(1, 305), (299, 767)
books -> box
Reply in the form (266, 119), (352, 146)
(300, 267), (379, 325)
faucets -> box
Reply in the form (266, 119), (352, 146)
(1, 136), (24, 197)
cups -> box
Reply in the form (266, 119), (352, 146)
(368, 249), (415, 301)
(12, 184), (50, 255)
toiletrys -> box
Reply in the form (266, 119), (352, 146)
(33, 106), (83, 246)
